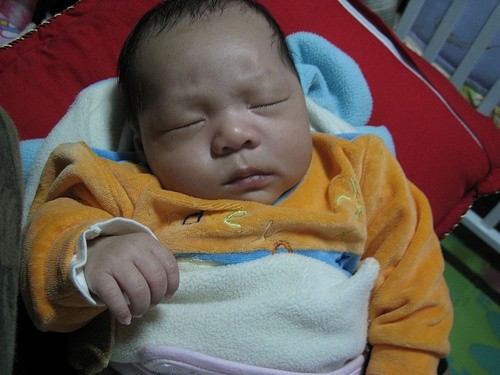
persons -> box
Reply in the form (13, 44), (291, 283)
(19, 0), (454, 374)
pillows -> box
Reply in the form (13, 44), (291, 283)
(1, 1), (500, 248)
